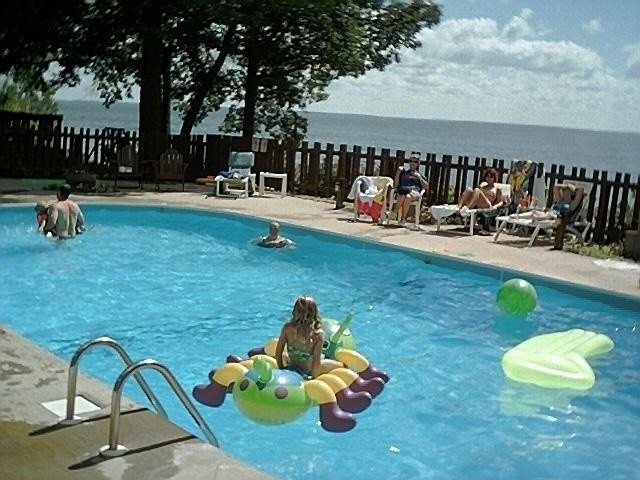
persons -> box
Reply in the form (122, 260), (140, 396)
(456, 168), (502, 211)
(44, 183), (85, 239)
(275, 295), (346, 380)
(260, 221), (286, 244)
(33, 200), (85, 237)
(389, 153), (429, 225)
(507, 182), (584, 222)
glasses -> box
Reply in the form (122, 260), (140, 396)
(410, 160), (418, 163)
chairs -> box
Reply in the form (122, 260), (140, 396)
(353, 175), (596, 247)
(215, 150), (256, 198)
(113, 144), (190, 193)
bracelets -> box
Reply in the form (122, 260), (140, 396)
(418, 175), (423, 181)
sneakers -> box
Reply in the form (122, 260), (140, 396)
(385, 211), (398, 220)
(394, 216), (406, 228)
(474, 229), (493, 236)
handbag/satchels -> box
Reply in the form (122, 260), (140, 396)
(399, 186), (412, 194)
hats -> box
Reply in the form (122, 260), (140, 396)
(563, 180), (577, 192)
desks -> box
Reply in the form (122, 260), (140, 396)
(259, 171), (288, 197)
